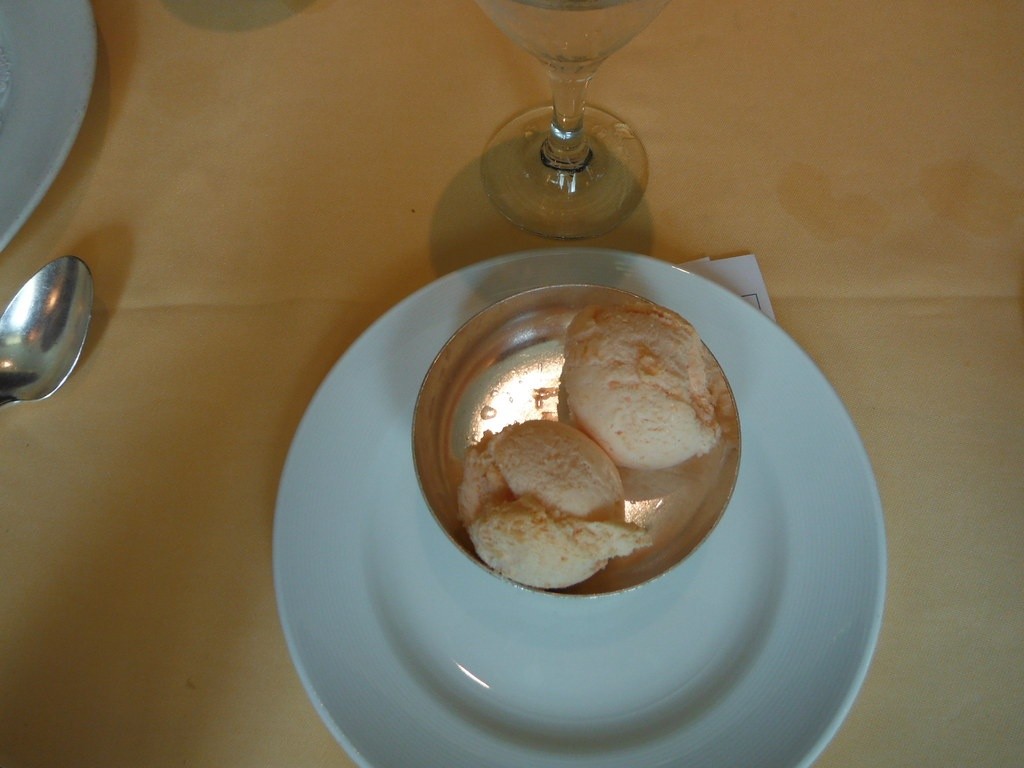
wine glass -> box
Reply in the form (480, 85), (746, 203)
(480, 0), (667, 239)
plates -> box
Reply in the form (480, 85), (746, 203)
(0, 0), (98, 254)
(272, 246), (887, 768)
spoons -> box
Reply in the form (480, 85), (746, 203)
(0, 255), (94, 407)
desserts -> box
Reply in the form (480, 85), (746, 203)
(571, 306), (723, 465)
(458, 420), (650, 589)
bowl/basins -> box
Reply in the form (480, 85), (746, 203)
(411, 284), (741, 598)
